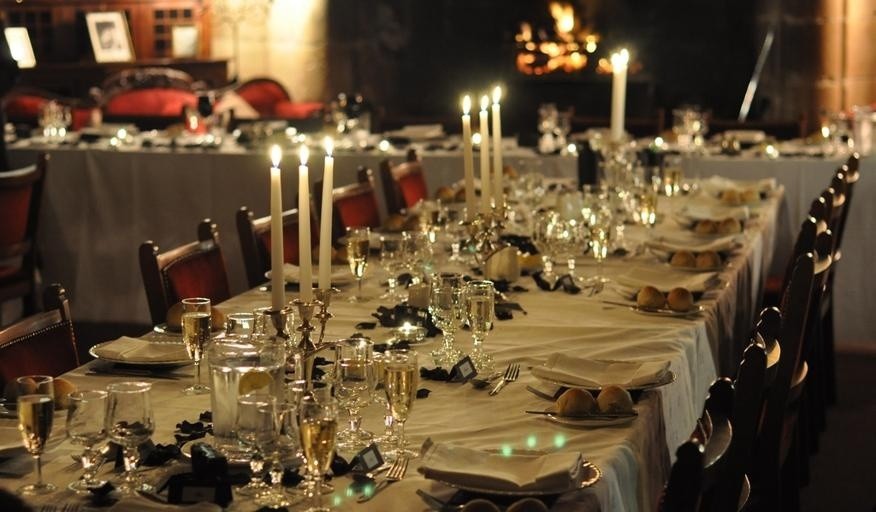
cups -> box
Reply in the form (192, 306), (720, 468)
(220, 308), (293, 336)
(199, 337), (285, 445)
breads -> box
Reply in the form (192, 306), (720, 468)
(669, 246), (723, 269)
(311, 243), (352, 263)
(636, 283), (694, 312)
(436, 186), (470, 204)
(719, 186), (762, 204)
(695, 216), (740, 237)
(5, 378), (77, 406)
(385, 213), (428, 232)
(166, 301), (226, 331)
(489, 164), (520, 179)
(556, 383), (633, 418)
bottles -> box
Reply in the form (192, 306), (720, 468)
(331, 93), (372, 135)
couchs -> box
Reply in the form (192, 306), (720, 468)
(4, 67), (327, 127)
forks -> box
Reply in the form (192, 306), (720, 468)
(40, 502), (83, 512)
(487, 366), (521, 396)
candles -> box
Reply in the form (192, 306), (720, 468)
(610, 48), (629, 145)
(460, 85), (507, 224)
(264, 136), (341, 313)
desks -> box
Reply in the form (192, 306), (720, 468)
(0, 173), (789, 512)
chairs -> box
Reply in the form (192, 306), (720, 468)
(655, 155), (862, 511)
(1, 153), (432, 409)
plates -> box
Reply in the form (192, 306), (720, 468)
(670, 175), (781, 241)
(87, 338), (187, 369)
(266, 261), (359, 287)
(147, 316), (225, 338)
(0, 395), (64, 461)
(539, 402), (637, 428)
(531, 364), (678, 392)
(776, 142), (834, 158)
(433, 445), (601, 495)
(677, 262), (735, 272)
(631, 301), (707, 318)
(181, 431), (289, 465)
(336, 233), (408, 251)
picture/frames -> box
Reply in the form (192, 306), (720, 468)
(84, 12), (137, 64)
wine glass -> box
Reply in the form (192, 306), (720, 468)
(182, 296), (214, 397)
(105, 381), (158, 499)
(232, 339), (418, 510)
(347, 160), (679, 365)
(14, 373), (59, 495)
(528, 103), (713, 156)
(65, 388), (110, 494)
